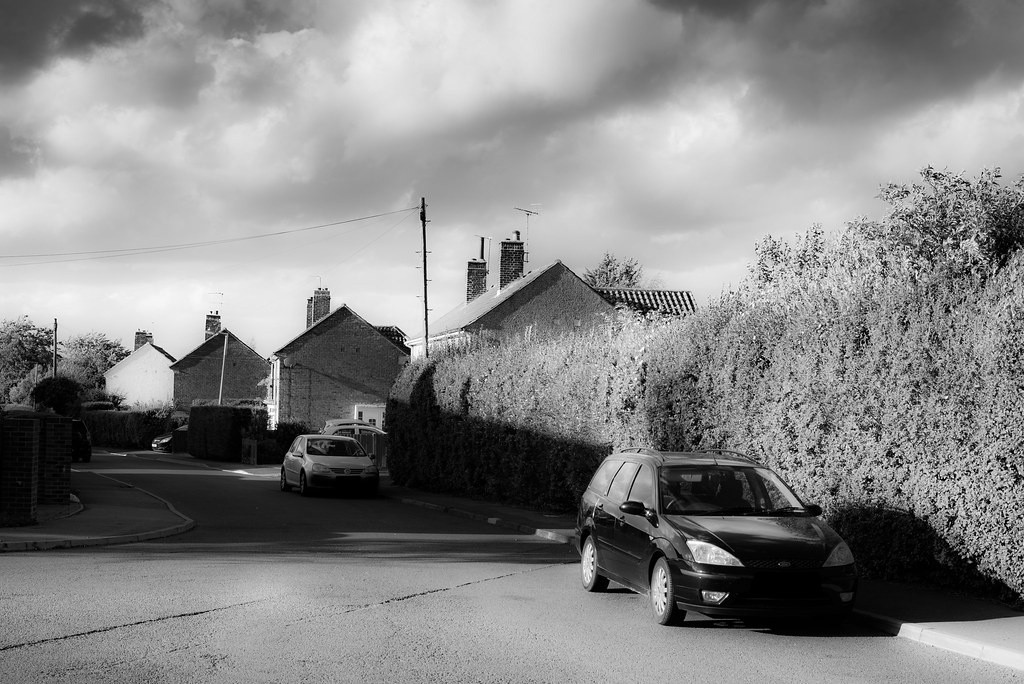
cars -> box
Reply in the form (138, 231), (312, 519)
(71, 419), (92, 462)
(280, 419), (388, 496)
(151, 432), (172, 452)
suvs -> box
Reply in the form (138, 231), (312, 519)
(574, 447), (858, 626)
